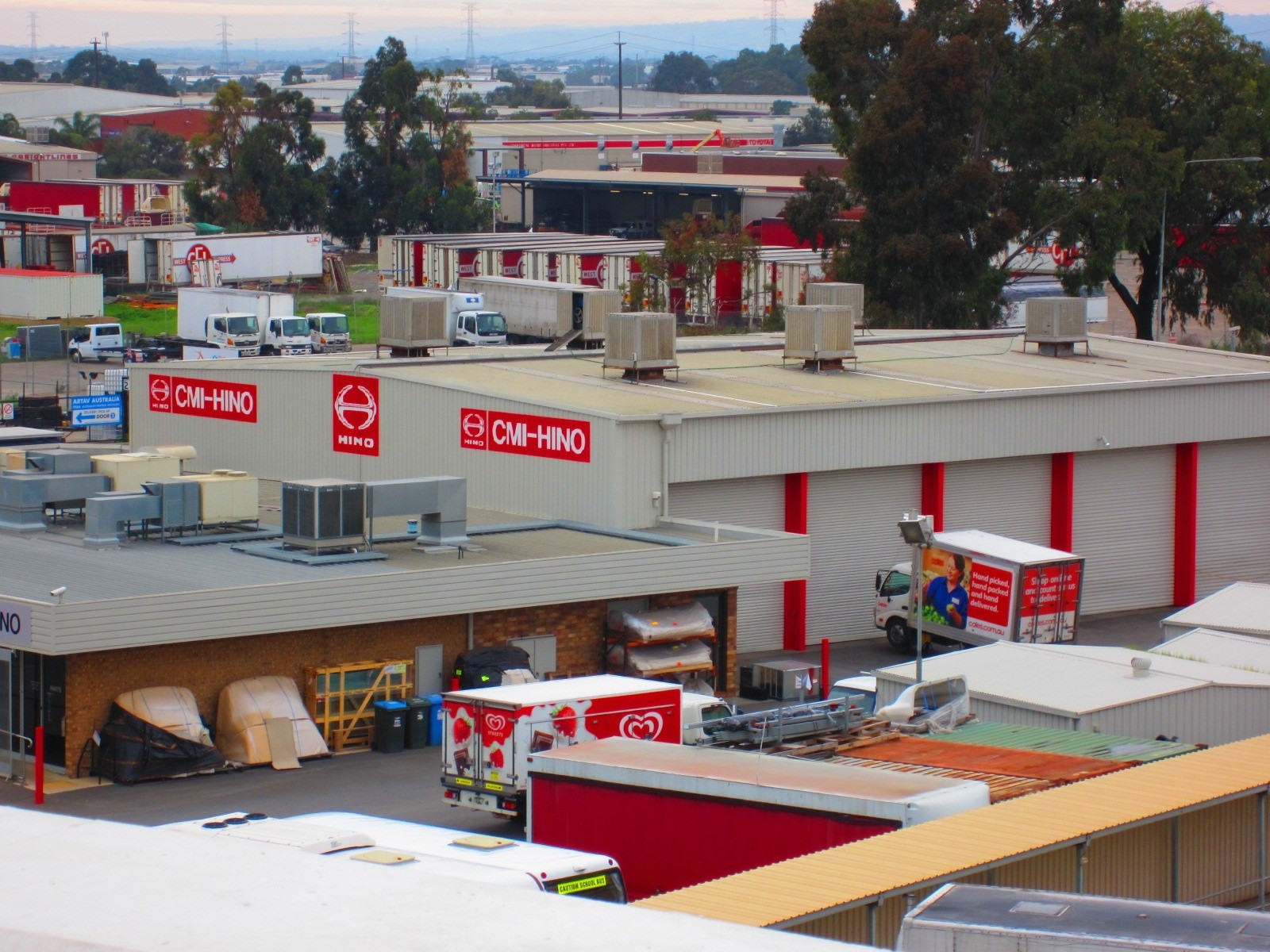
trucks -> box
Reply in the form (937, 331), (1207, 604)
(430, 673), (751, 821)
(306, 312), (351, 355)
(65, 323), (170, 361)
(174, 285), (312, 357)
(132, 313), (260, 359)
(869, 529), (1087, 655)
(383, 284), (508, 349)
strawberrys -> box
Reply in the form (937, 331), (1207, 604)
(489, 746), (504, 767)
(549, 702), (577, 738)
(454, 717), (472, 744)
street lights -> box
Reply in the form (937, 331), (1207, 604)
(1159, 155), (1264, 345)
(899, 512), (935, 682)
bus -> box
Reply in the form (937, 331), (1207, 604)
(168, 810), (623, 912)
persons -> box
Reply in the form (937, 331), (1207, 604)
(915, 553), (970, 630)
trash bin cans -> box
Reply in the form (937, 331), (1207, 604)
(426, 695), (443, 745)
(372, 699), (412, 754)
(399, 698), (430, 749)
